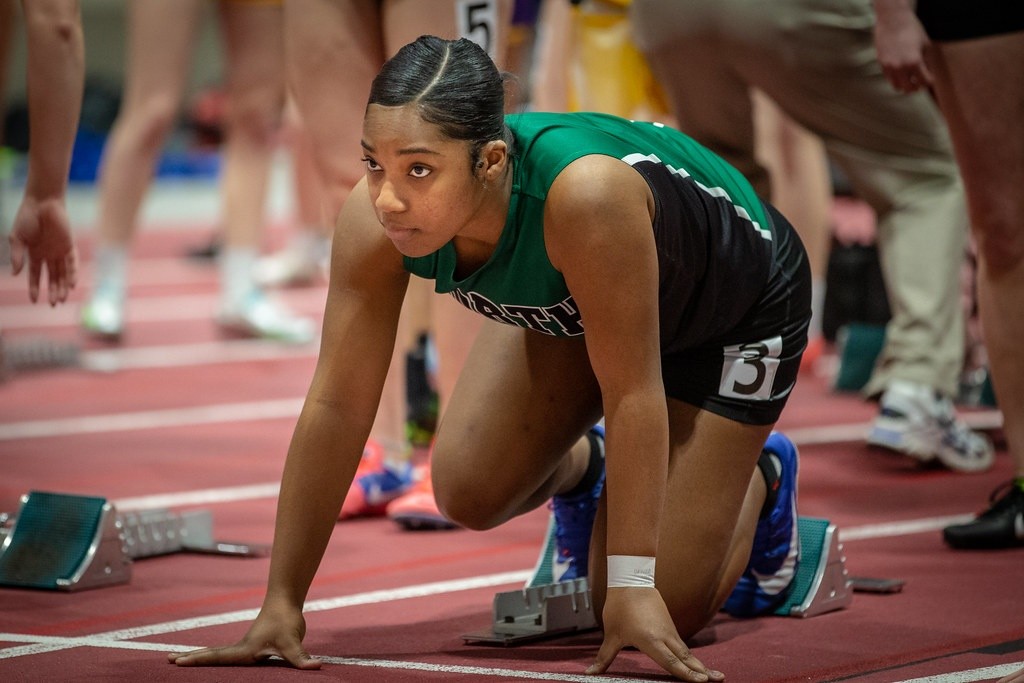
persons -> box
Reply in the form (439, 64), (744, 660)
(910, 2), (1022, 556)
(624, 0), (997, 474)
(529, 3), (685, 143)
(162, 26), (813, 683)
(1, 0), (87, 313)
(80, 0), (315, 346)
(256, 102), (338, 297)
(751, 79), (838, 370)
(279, 0), (514, 536)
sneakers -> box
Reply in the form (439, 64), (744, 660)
(552, 425), (606, 579)
(722, 431), (800, 620)
(340, 438), (460, 530)
(866, 376), (997, 476)
(80, 250), (126, 338)
(219, 253), (313, 340)
(944, 486), (1024, 550)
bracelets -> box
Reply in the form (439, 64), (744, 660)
(605, 553), (656, 590)
